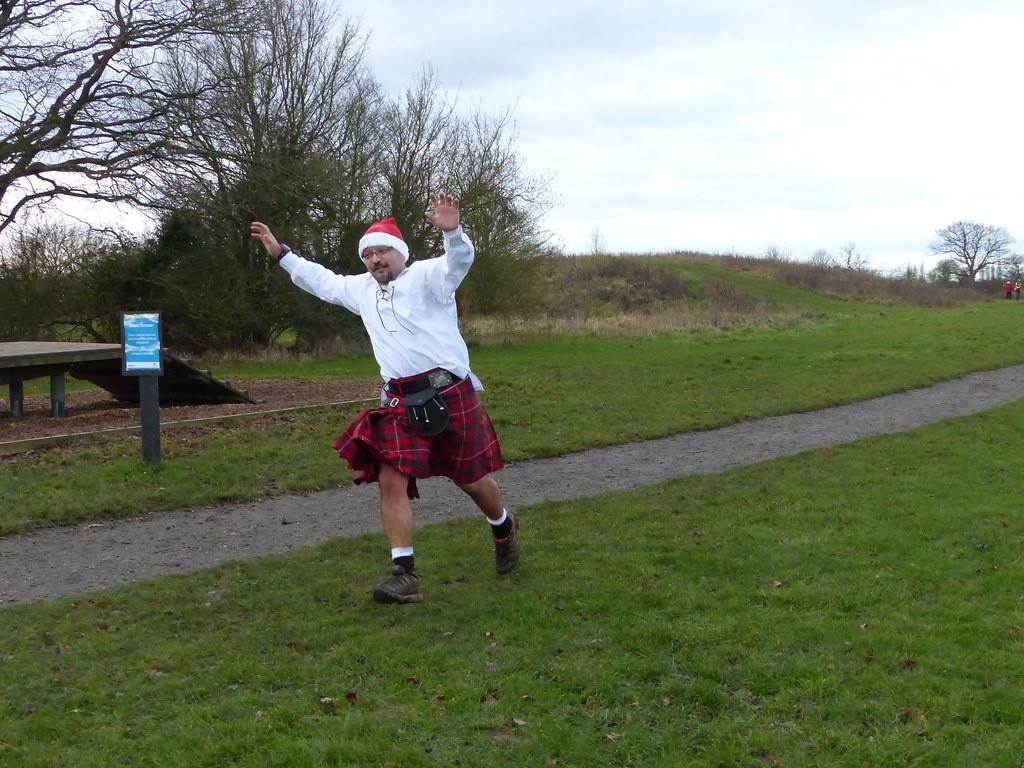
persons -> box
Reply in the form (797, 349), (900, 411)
(1003, 278), (1022, 301)
(250, 191), (520, 602)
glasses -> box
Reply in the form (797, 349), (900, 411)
(362, 247), (390, 260)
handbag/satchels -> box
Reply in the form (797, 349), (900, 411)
(404, 388), (449, 436)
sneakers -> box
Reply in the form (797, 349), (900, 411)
(493, 510), (520, 575)
(372, 565), (423, 603)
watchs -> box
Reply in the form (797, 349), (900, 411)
(278, 244), (290, 261)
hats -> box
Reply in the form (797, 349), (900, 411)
(358, 217), (410, 263)
